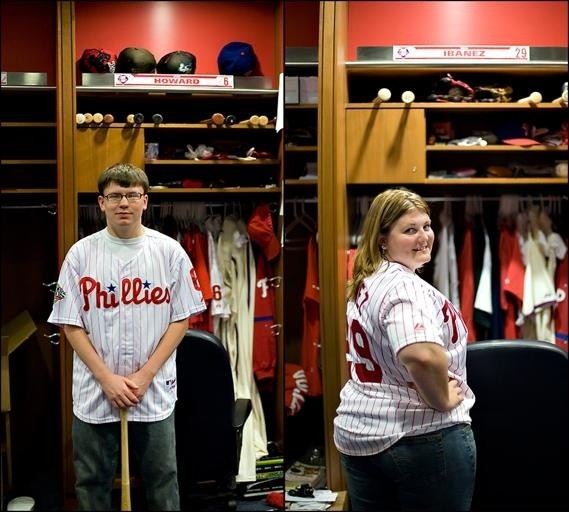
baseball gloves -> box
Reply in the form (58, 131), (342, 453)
(428, 74), (513, 103)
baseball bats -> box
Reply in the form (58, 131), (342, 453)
(371, 88), (415, 104)
(120, 409), (131, 512)
(75, 112), (277, 124)
(517, 89), (568, 103)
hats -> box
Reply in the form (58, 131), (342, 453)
(218, 42), (255, 74)
(80, 47), (196, 76)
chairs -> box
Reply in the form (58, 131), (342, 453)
(176, 328), (253, 512)
(467, 339), (569, 512)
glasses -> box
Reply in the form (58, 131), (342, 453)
(104, 193), (144, 202)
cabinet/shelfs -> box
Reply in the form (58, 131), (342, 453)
(341, 62), (569, 185)
(282, 103), (319, 185)
(74, 85), (281, 198)
(0, 85), (58, 194)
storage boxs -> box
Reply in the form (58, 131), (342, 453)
(1, 310), (36, 411)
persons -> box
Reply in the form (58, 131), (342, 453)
(333, 188), (477, 512)
(47, 164), (207, 512)
